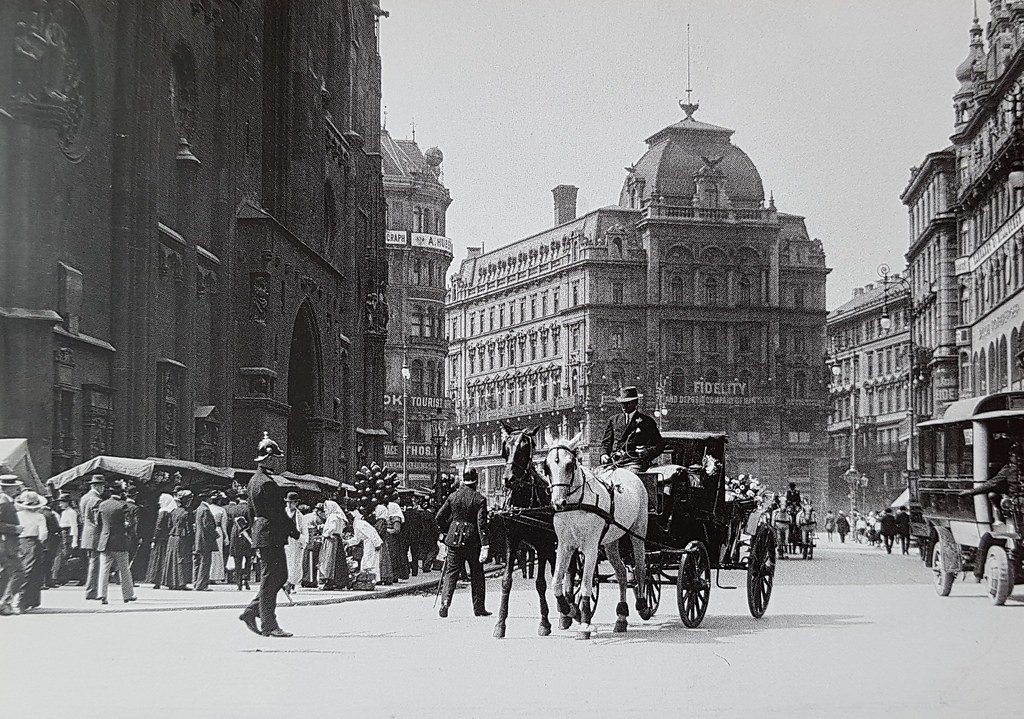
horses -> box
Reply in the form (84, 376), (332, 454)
(493, 419), (652, 640)
(771, 490), (817, 560)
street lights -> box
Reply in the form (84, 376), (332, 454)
(878, 273), (916, 472)
(846, 467), (868, 542)
(429, 405), (450, 509)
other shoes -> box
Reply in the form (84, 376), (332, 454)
(475, 610), (492, 616)
(239, 612), (262, 635)
(124, 595), (137, 603)
(101, 598), (108, 604)
(262, 625), (293, 637)
(439, 602), (448, 618)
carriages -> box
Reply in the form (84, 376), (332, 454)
(486, 417), (780, 641)
(769, 497), (820, 561)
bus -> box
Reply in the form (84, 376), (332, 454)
(904, 388), (1024, 607)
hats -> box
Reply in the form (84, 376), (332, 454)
(0, 474), (23, 486)
(315, 502), (324, 511)
(55, 493), (71, 502)
(87, 474), (108, 484)
(284, 492), (301, 502)
(172, 486), (249, 501)
(15, 490), (48, 509)
(618, 387), (643, 402)
(105, 483), (128, 493)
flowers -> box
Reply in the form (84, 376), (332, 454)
(704, 454), (723, 476)
(725, 472), (768, 510)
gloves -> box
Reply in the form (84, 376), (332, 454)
(478, 545), (490, 563)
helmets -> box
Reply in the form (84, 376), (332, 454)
(463, 460), (477, 485)
(254, 431), (284, 461)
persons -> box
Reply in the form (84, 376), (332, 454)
(825, 510), (882, 546)
(0, 475), (79, 616)
(96, 484), (137, 605)
(116, 485), (140, 586)
(600, 385), (665, 474)
(768, 495), (781, 522)
(145, 486), (254, 590)
(240, 439), (301, 637)
(435, 467), (492, 616)
(958, 443), (1024, 548)
(881, 508), (896, 553)
(896, 506), (913, 554)
(281, 492), (384, 593)
(375, 494), (440, 586)
(481, 511), (507, 565)
(80, 474), (108, 599)
(786, 482), (802, 514)
